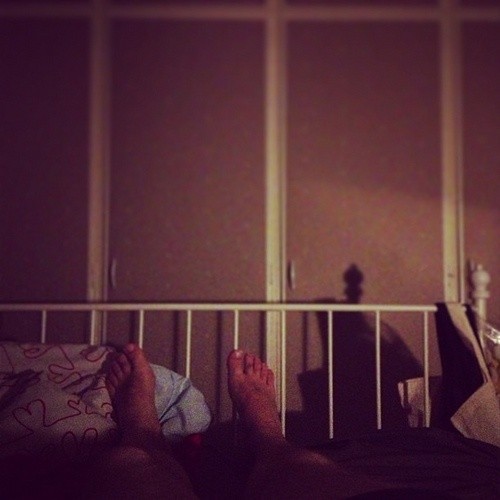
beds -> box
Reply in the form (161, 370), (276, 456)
(0, 297), (500, 500)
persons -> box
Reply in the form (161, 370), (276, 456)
(91, 340), (367, 500)
